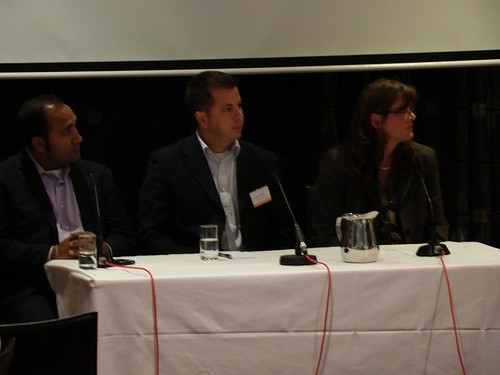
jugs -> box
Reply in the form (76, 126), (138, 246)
(336, 211), (379, 263)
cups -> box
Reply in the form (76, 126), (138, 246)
(78, 234), (97, 270)
(199, 225), (218, 261)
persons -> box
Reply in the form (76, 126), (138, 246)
(304, 77), (451, 248)
(138, 71), (296, 254)
(0, 94), (138, 326)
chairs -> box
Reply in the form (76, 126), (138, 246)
(0, 311), (97, 375)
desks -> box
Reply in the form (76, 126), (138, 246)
(44, 241), (500, 375)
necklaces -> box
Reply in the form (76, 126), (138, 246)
(378, 163), (394, 172)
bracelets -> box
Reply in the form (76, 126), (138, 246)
(53, 244), (59, 257)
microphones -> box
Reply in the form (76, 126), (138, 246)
(265, 167), (317, 265)
(413, 166), (451, 257)
(85, 169), (135, 267)
